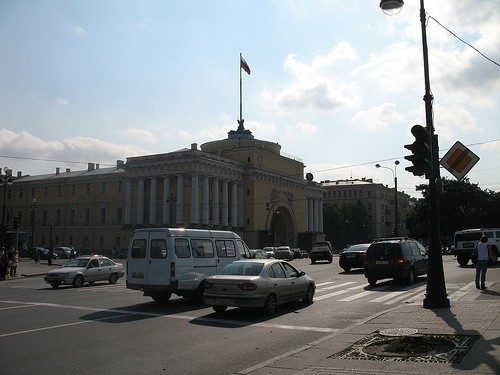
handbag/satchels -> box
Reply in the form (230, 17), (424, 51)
(471, 243), (478, 264)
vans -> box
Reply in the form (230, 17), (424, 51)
(124, 227), (257, 303)
(452, 227), (500, 264)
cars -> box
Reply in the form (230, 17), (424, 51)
(249, 245), (309, 261)
(309, 246), (334, 264)
(42, 255), (124, 288)
(19, 246), (59, 260)
(200, 258), (316, 316)
(52, 247), (77, 258)
(339, 243), (370, 272)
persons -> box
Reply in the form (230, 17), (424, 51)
(0, 247), (17, 281)
(475, 235), (491, 289)
(70, 247), (81, 260)
(32, 248), (40, 264)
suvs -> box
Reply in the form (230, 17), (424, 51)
(364, 237), (428, 285)
(316, 241), (334, 252)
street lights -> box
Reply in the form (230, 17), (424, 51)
(31, 199), (37, 246)
(375, 160), (400, 238)
(380, 0), (452, 308)
(0, 167), (9, 246)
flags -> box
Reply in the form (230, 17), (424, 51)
(241, 56), (251, 75)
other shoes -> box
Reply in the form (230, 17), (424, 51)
(481, 287), (487, 289)
(476, 285), (480, 289)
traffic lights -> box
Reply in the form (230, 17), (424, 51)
(404, 142), (423, 176)
(410, 125), (432, 171)
(3, 224), (8, 233)
(13, 216), (20, 230)
